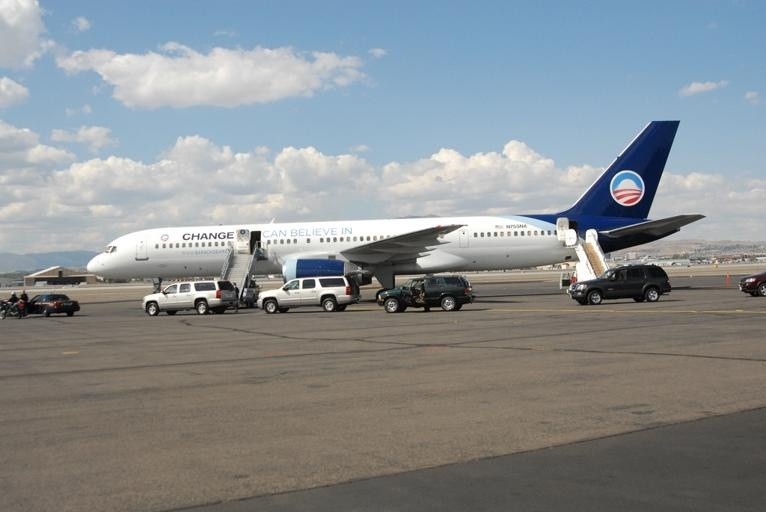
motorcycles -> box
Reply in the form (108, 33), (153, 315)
(0, 299), (23, 321)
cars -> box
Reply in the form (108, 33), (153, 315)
(26, 294), (82, 318)
(738, 269), (765, 297)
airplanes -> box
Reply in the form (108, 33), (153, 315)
(85, 120), (706, 306)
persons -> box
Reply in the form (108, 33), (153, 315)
(233, 282), (239, 313)
(8, 290), (29, 319)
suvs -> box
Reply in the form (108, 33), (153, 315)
(378, 272), (474, 314)
(254, 274), (361, 314)
(142, 279), (237, 314)
(567, 264), (672, 305)
